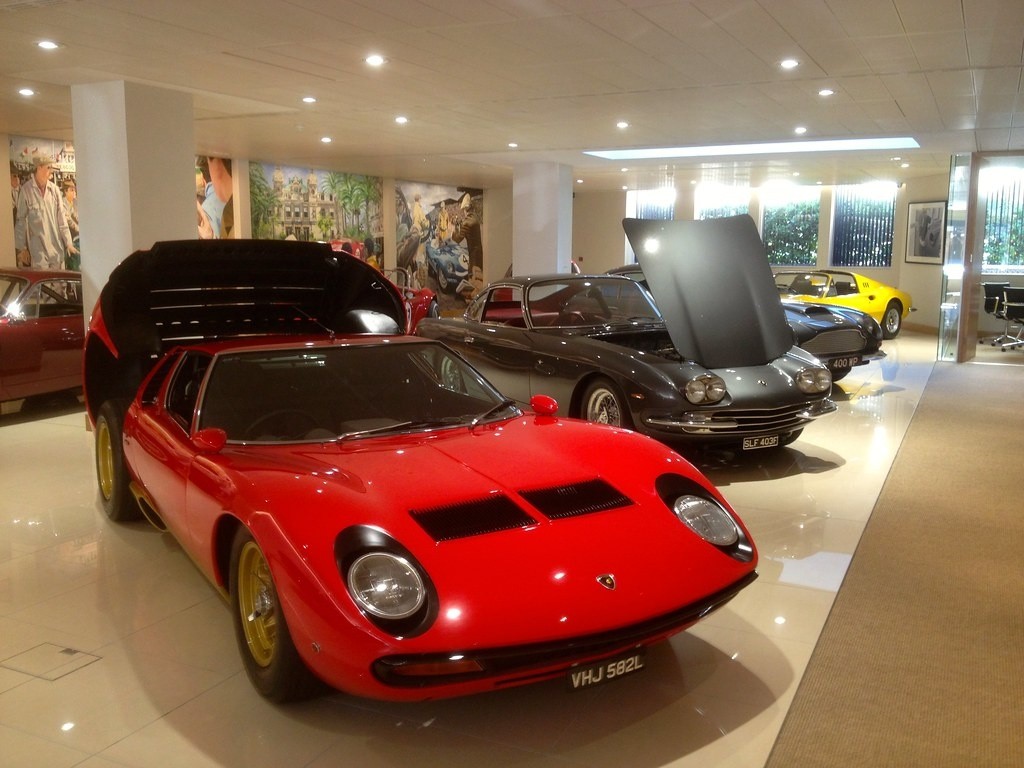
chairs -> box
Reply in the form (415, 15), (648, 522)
(979, 282), (1024, 352)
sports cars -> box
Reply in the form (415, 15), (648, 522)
(80, 235), (761, 709)
(408, 214), (918, 463)
(0, 268), (87, 405)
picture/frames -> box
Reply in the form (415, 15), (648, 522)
(905, 201), (948, 266)
